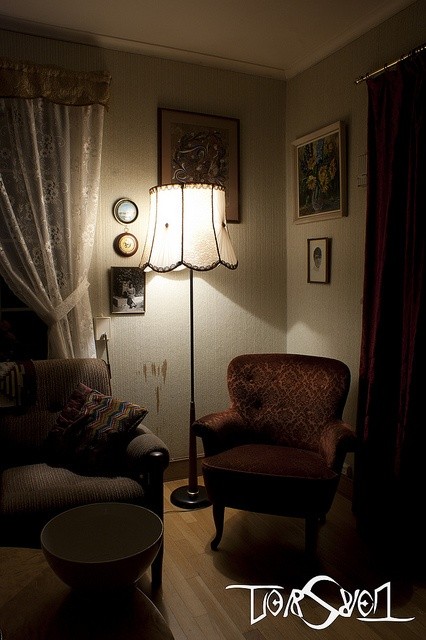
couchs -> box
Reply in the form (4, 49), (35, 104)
(0, 358), (170, 590)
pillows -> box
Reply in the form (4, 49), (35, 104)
(44, 380), (147, 475)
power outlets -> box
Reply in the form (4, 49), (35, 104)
(95, 316), (111, 341)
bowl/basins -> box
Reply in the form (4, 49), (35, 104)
(39, 502), (164, 601)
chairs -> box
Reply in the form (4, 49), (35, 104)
(192, 352), (357, 562)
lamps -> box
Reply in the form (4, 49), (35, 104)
(134, 182), (239, 513)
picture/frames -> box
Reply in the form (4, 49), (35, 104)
(307, 238), (331, 284)
(114, 198), (139, 227)
(114, 233), (139, 257)
(157, 106), (241, 223)
(110, 265), (146, 314)
(290, 121), (347, 225)
